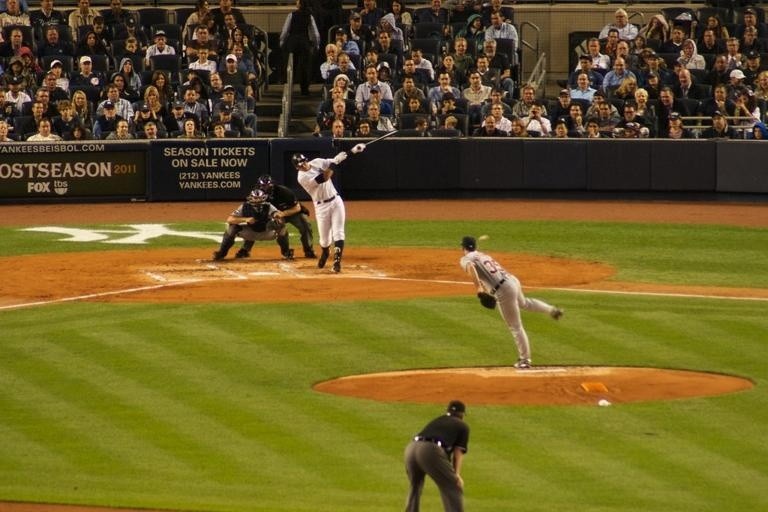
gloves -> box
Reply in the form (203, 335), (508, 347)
(332, 151), (348, 164)
(351, 143), (366, 154)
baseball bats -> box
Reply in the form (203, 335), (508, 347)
(358, 130), (399, 149)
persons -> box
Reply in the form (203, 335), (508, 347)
(195, 189), (285, 266)
(1, 0), (266, 142)
(404, 399), (469, 512)
(258, 174), (318, 260)
(314, 0), (552, 141)
(548, 1), (768, 141)
(280, 1), (320, 95)
(459, 235), (563, 366)
(292, 142), (366, 274)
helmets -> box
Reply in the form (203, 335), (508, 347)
(447, 400), (467, 416)
(292, 153), (308, 169)
(249, 190), (267, 202)
(258, 175), (272, 186)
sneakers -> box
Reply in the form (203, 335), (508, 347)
(281, 247), (295, 259)
(305, 246), (317, 259)
(514, 359), (532, 369)
(332, 260), (342, 271)
(212, 246), (228, 260)
(235, 248), (250, 258)
(550, 307), (564, 320)
(318, 253), (329, 269)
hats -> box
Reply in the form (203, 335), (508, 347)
(460, 236), (477, 251)
(6, 31), (240, 114)
(335, 10), (512, 135)
(555, 8), (767, 136)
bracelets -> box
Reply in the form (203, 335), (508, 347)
(329, 163), (336, 170)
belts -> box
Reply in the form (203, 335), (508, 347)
(412, 435), (449, 451)
(488, 274), (508, 294)
(317, 192), (340, 204)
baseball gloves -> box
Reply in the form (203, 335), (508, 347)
(478, 292), (496, 309)
(270, 213), (284, 230)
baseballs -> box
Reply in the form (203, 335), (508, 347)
(479, 236), (489, 241)
(598, 400), (608, 406)
